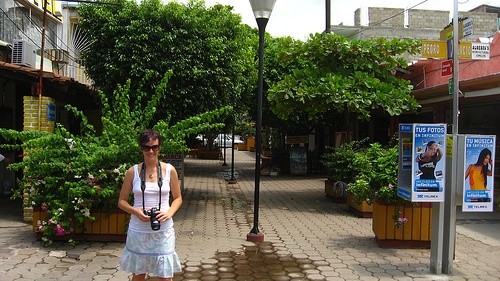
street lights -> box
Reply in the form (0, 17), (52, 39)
(247, 0), (278, 242)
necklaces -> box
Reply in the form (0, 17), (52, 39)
(149, 174), (153, 178)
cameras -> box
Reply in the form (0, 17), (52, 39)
(143, 207), (161, 230)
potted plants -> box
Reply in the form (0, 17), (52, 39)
(325, 140), (400, 219)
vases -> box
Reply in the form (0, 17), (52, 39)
(34, 204), (132, 243)
(373, 197), (433, 250)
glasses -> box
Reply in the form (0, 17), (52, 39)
(140, 144), (160, 151)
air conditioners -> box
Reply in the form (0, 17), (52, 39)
(51, 49), (69, 64)
(11, 39), (36, 69)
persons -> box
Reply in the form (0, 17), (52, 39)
(415, 141), (443, 192)
(465, 148), (494, 202)
(118, 129), (183, 281)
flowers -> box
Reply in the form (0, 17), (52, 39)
(365, 176), (421, 228)
(29, 162), (133, 248)
